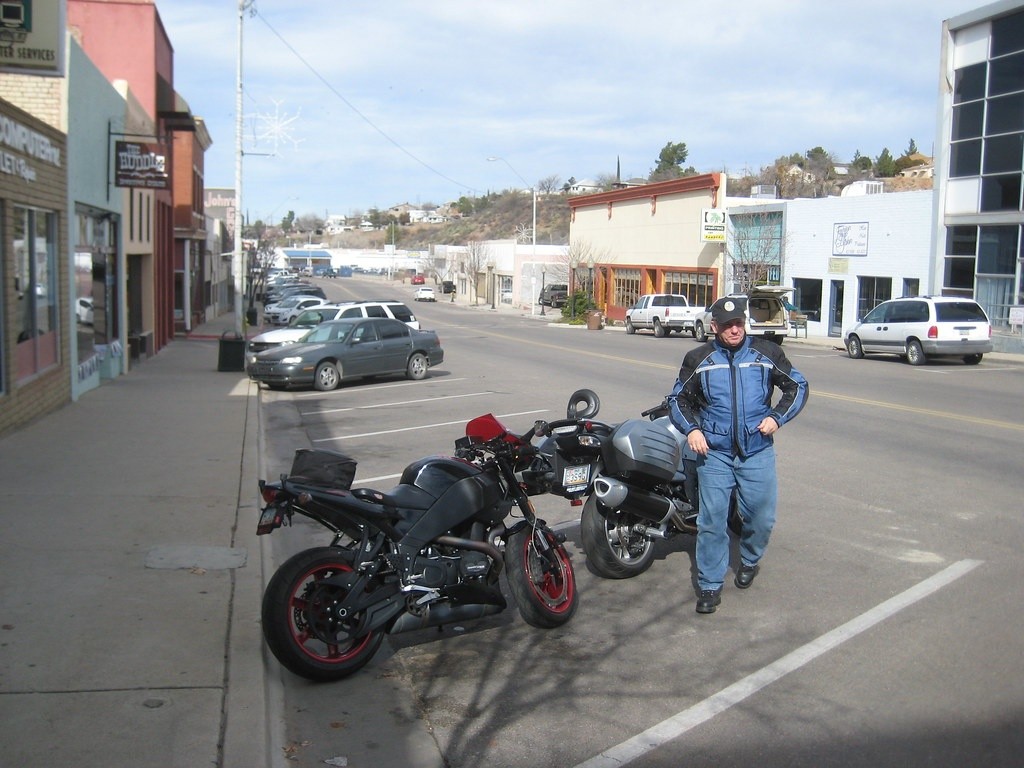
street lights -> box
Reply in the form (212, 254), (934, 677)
(491, 269), (496, 309)
(234, 151), (277, 338)
(540, 265), (547, 316)
(486, 156), (537, 246)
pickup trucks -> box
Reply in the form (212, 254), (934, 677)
(624, 293), (706, 339)
(538, 284), (568, 308)
(439, 281), (456, 293)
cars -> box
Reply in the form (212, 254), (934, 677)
(17, 282), (46, 298)
(77, 298), (97, 324)
(411, 275), (424, 285)
(501, 289), (512, 302)
(858, 299), (885, 318)
(323, 272), (336, 279)
(414, 287), (435, 302)
(248, 317), (445, 392)
(350, 265), (393, 276)
(260, 268), (332, 326)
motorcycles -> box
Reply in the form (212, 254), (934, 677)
(534, 388), (748, 579)
(255, 412), (577, 684)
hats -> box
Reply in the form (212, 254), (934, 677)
(712, 296), (745, 323)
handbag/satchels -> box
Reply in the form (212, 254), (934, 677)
(287, 449), (357, 491)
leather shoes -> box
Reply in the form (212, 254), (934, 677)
(734, 559), (756, 588)
(696, 590), (721, 612)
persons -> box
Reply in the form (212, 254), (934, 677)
(668, 294), (810, 614)
(782, 297), (798, 311)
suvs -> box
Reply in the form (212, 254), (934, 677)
(247, 299), (421, 363)
(843, 295), (994, 366)
(693, 286), (797, 346)
